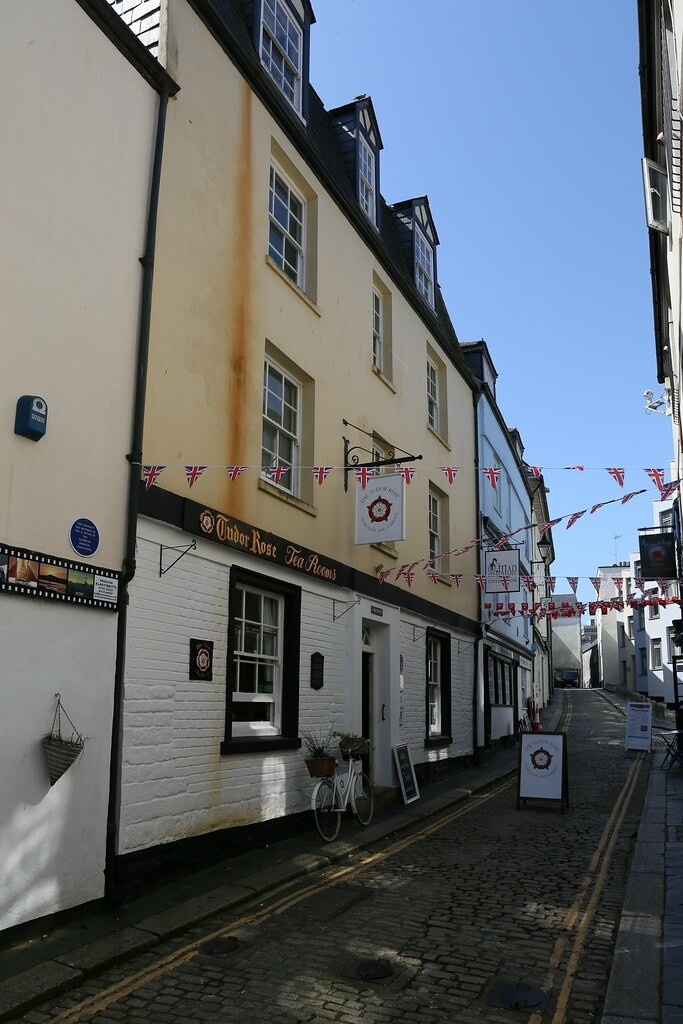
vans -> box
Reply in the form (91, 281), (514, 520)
(558, 671), (581, 689)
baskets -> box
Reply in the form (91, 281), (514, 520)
(41, 700), (86, 786)
(303, 755), (335, 777)
(339, 737), (368, 760)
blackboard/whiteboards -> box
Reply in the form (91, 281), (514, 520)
(393, 742), (420, 803)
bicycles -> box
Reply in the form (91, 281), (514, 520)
(304, 738), (374, 843)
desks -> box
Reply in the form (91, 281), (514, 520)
(656, 729), (682, 770)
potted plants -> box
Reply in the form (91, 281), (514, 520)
(298, 721), (337, 778)
(331, 730), (376, 761)
(40, 728), (96, 787)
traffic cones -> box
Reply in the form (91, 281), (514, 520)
(532, 707), (542, 732)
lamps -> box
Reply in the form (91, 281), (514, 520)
(531, 531), (553, 565)
